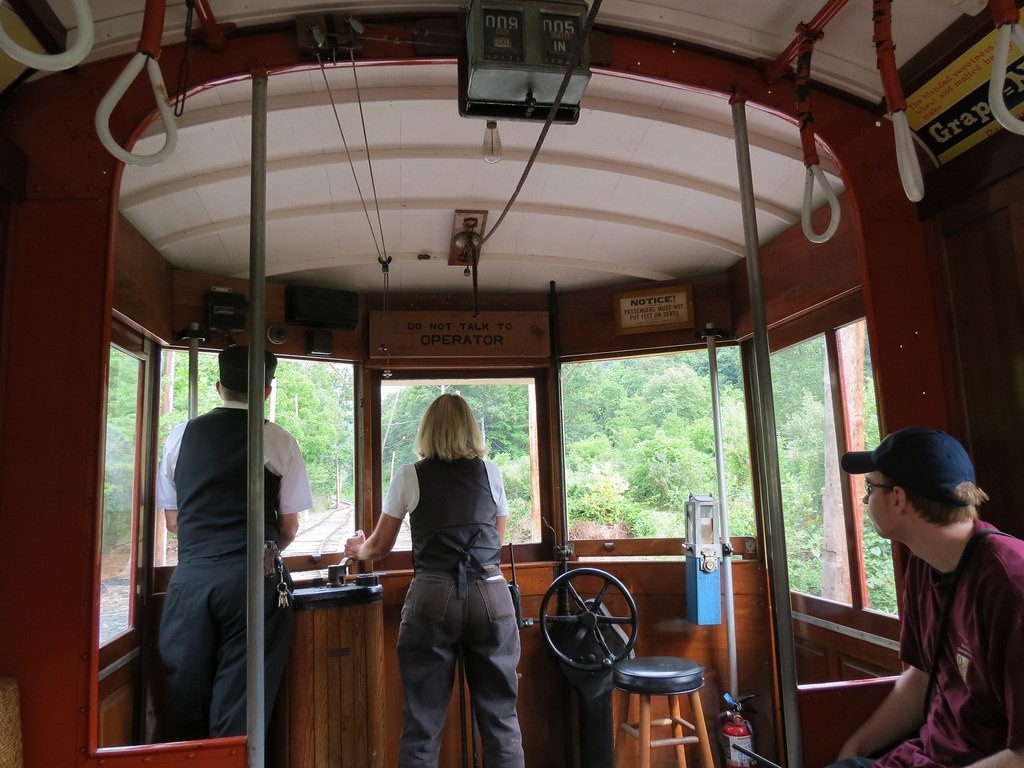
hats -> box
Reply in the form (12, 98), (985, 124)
(219, 347), (277, 392)
(841, 428), (976, 508)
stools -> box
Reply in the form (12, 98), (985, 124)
(611, 657), (715, 768)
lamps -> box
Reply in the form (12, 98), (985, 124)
(481, 121), (504, 163)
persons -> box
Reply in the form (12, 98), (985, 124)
(155, 345), (313, 768)
(343, 394), (525, 768)
(829, 426), (1024, 768)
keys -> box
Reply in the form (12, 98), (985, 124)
(278, 588), (295, 608)
(263, 548), (277, 577)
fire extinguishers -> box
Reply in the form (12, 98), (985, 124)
(715, 692), (762, 768)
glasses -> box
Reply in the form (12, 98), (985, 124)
(864, 480), (892, 497)
